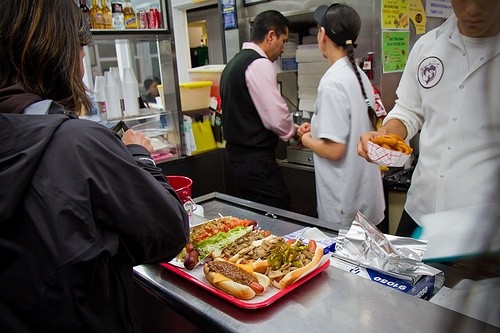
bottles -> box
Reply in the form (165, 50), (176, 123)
(148, 8), (158, 29)
(101, 0), (113, 30)
(135, 6), (148, 29)
(79, 0), (92, 29)
(110, 0), (124, 30)
(90, 0), (103, 29)
(123, 0), (137, 29)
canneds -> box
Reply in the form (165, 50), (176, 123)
(147, 8), (161, 30)
(136, 8), (147, 29)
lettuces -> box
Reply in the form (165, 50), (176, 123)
(196, 224), (254, 260)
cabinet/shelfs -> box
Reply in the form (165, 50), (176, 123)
(75, 0), (188, 165)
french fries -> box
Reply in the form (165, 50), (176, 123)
(371, 133), (414, 155)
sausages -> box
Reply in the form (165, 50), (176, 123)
(183, 249), (199, 270)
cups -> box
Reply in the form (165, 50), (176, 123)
(104, 71), (122, 119)
(93, 75), (107, 119)
(123, 67), (140, 118)
(109, 67), (123, 100)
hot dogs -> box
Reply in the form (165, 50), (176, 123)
(269, 239), (324, 291)
(236, 236), (300, 272)
(203, 256), (270, 300)
(211, 230), (274, 261)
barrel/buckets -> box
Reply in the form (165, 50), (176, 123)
(165, 175), (192, 206)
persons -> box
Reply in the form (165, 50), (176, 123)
(2, 0), (191, 331)
(298, 2), (389, 230)
(143, 77), (161, 103)
(356, 0), (499, 261)
(219, 10), (300, 209)
